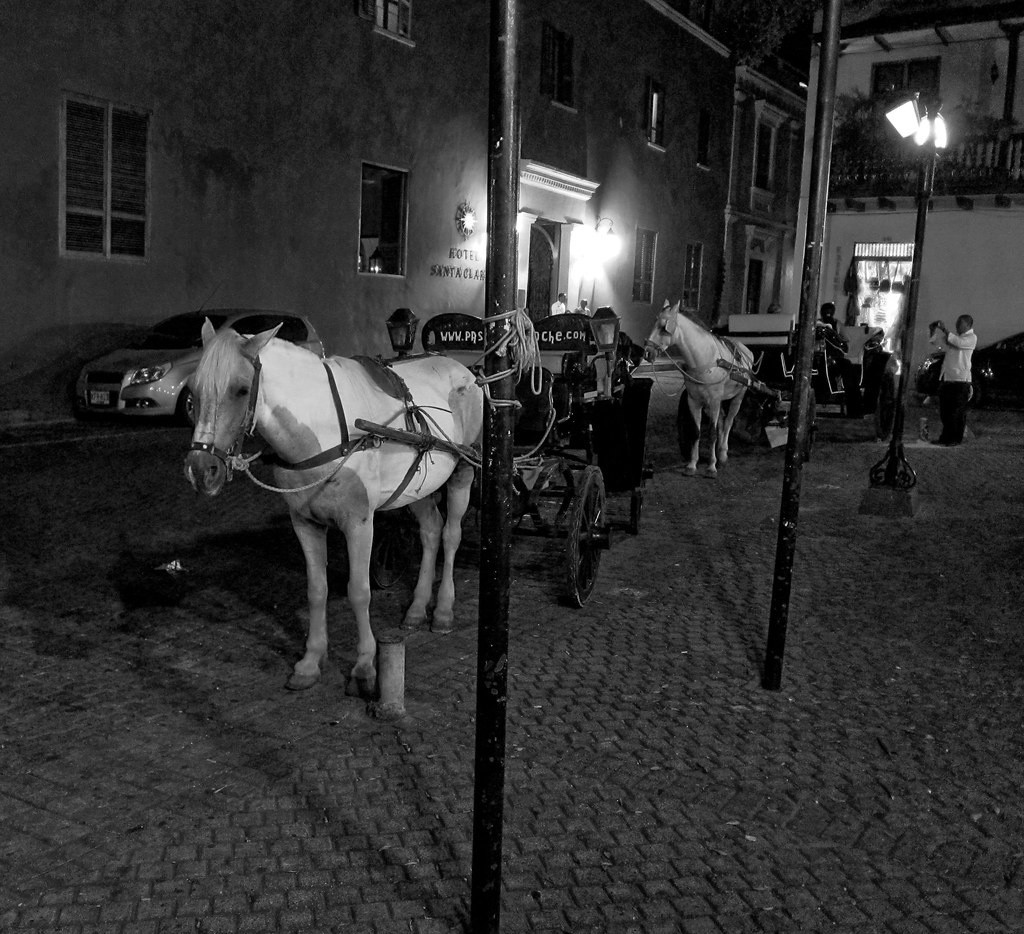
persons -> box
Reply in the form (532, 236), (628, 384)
(574, 300), (591, 317)
(767, 302), (783, 314)
(550, 292), (571, 317)
(928, 314), (978, 446)
(818, 303), (849, 353)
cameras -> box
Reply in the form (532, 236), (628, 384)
(932, 321), (940, 327)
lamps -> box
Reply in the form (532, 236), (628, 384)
(589, 307), (622, 352)
(385, 308), (420, 352)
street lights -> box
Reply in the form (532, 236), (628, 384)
(871, 89), (962, 488)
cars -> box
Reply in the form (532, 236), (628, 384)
(917, 332), (1024, 403)
(72, 307), (328, 425)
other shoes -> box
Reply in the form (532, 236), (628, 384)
(930, 436), (963, 446)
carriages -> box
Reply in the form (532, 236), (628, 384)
(183, 312), (653, 698)
(644, 298), (892, 478)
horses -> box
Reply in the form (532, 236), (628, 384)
(643, 300), (756, 482)
(178, 317), (484, 704)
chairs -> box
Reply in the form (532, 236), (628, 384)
(841, 326), (884, 387)
(533, 313), (603, 435)
(728, 313), (795, 377)
(420, 312), (485, 378)
